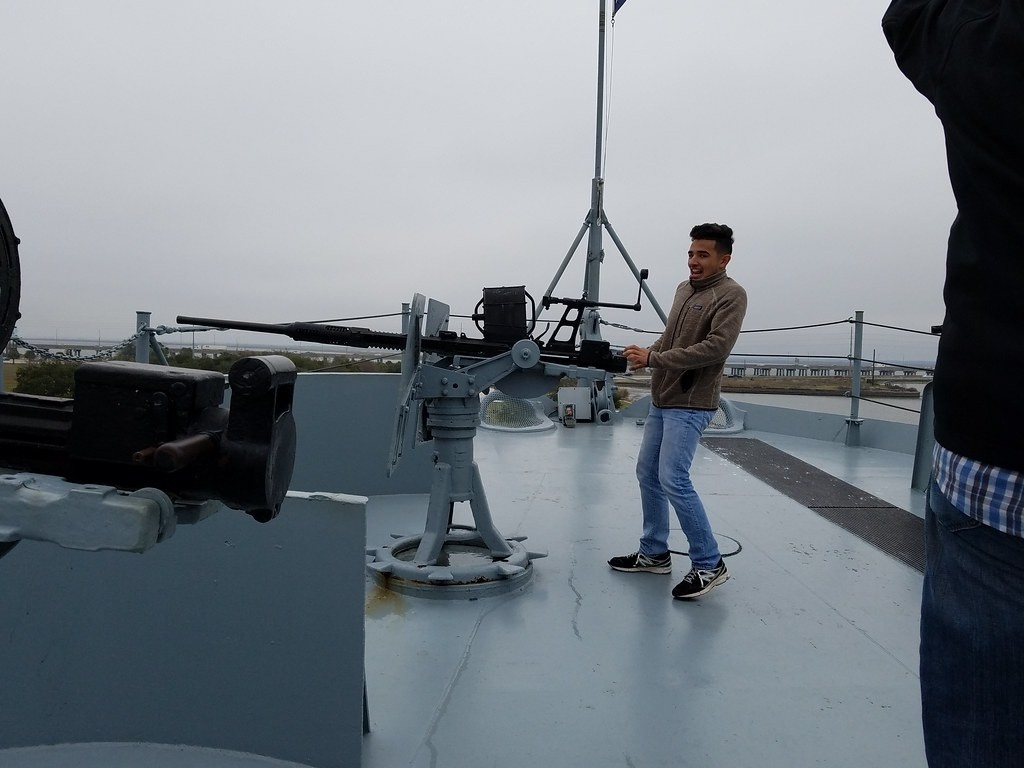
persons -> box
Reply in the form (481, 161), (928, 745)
(606, 220), (748, 601)
(881, 0), (1024, 768)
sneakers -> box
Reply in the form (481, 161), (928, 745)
(672, 557), (729, 598)
(607, 551), (672, 575)
